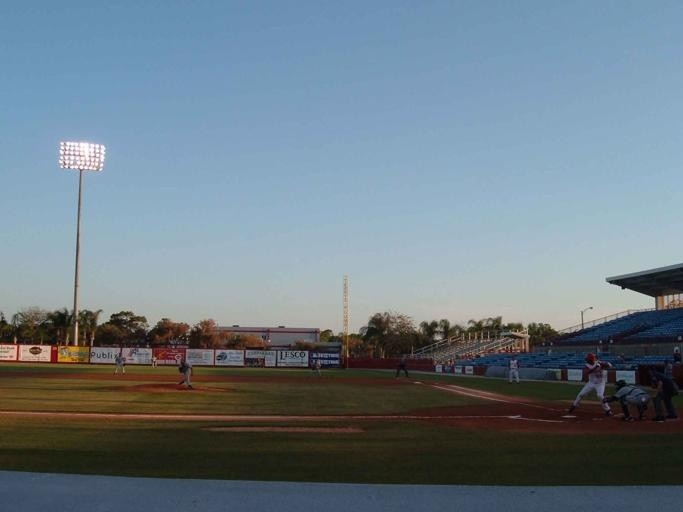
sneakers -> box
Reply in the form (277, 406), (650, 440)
(652, 416), (677, 422)
(606, 409), (646, 422)
(564, 410), (576, 415)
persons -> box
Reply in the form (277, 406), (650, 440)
(315, 358), (322, 377)
(395, 353), (409, 377)
(114, 352), (126, 375)
(151, 354), (158, 368)
(178, 360), (193, 389)
(569, 353), (613, 417)
(603, 379), (651, 422)
(508, 355), (520, 384)
(648, 365), (680, 422)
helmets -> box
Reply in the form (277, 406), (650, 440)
(614, 380), (626, 392)
(584, 353), (595, 361)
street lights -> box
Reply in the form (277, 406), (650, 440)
(581, 307), (593, 329)
(58, 141), (107, 346)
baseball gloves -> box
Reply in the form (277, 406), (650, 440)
(603, 396), (615, 403)
(123, 363), (126, 366)
